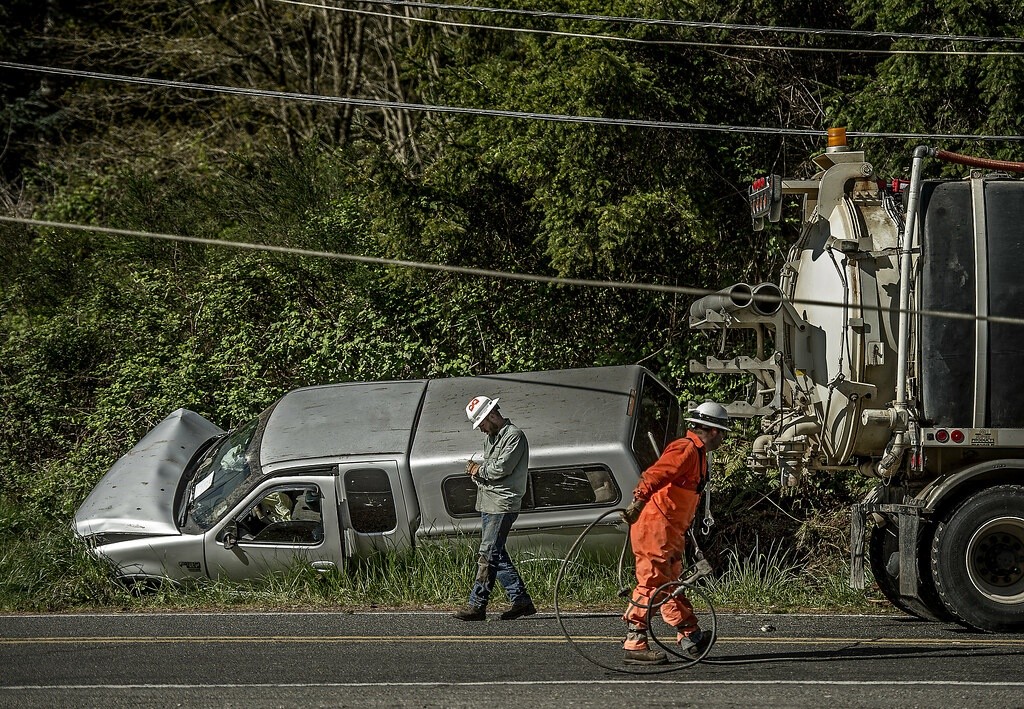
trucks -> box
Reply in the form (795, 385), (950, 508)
(688, 123), (1023, 635)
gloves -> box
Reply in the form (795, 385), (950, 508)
(464, 460), (480, 477)
(620, 497), (646, 524)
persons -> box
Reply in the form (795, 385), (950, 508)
(453, 395), (537, 621)
(620, 402), (732, 665)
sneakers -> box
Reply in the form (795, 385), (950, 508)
(621, 648), (668, 665)
(452, 604), (487, 621)
(688, 630), (716, 658)
(501, 595), (536, 619)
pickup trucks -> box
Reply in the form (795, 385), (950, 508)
(66, 363), (689, 597)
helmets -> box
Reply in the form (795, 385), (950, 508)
(683, 402), (733, 431)
(465, 396), (500, 430)
